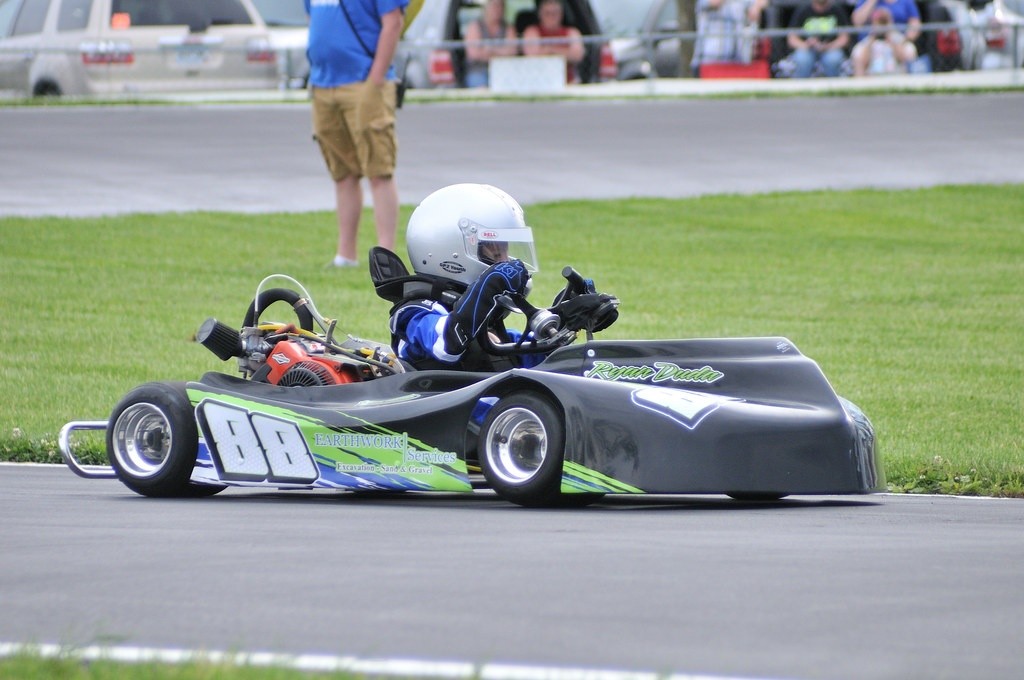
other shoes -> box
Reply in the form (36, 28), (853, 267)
(334, 255), (358, 267)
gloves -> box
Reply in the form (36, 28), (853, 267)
(549, 279), (616, 332)
(454, 259), (529, 339)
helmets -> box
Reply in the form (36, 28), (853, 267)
(407, 183), (540, 298)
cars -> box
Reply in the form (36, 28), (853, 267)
(593, 1), (681, 79)
(254, 0), (311, 85)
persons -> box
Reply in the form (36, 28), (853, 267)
(304, 0), (412, 267)
(388, 183), (594, 422)
(464, 0), (922, 85)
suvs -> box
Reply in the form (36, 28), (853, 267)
(763, 2), (983, 77)
(0, 0), (280, 101)
(391, 1), (617, 88)
